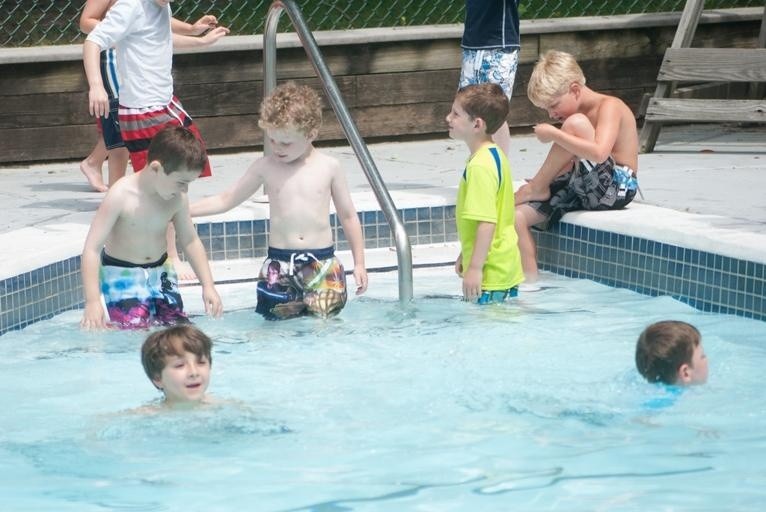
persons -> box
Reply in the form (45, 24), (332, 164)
(515, 48), (639, 284)
(446, 83), (526, 305)
(78, 0), (219, 192)
(457, 0), (521, 158)
(111, 324), (245, 414)
(634, 319), (710, 389)
(187, 80), (368, 321)
(258, 260), (282, 293)
(82, 0), (230, 178)
(80, 124), (222, 329)
